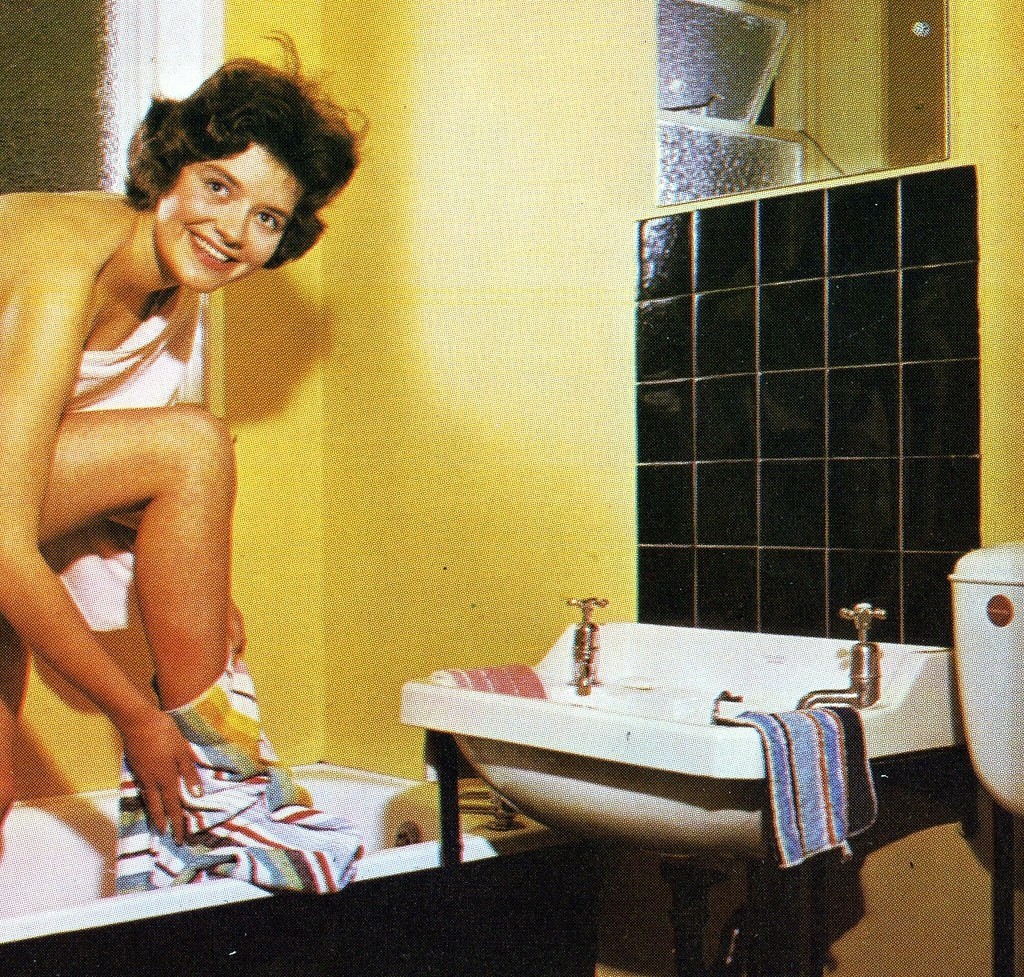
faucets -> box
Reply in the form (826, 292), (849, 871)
(562, 596), (610, 698)
(794, 599), (887, 710)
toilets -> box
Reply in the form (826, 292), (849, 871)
(945, 540), (1024, 822)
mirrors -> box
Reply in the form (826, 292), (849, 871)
(655, 0), (950, 207)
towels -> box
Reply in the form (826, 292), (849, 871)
(712, 703), (880, 871)
(112, 632), (367, 898)
(428, 664), (547, 701)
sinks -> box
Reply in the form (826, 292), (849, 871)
(398, 619), (965, 871)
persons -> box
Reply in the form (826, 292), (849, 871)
(0, 30), (360, 849)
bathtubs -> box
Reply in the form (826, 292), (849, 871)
(0, 761), (597, 977)
